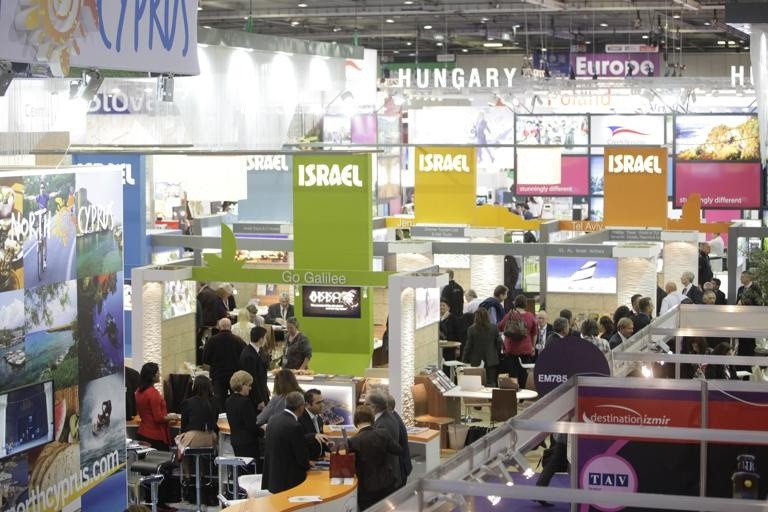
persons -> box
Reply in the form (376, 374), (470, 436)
(499, 294), (539, 388)
(281, 316), (312, 370)
(737, 299), (756, 380)
(681, 270), (702, 304)
(698, 243), (713, 286)
(531, 433), (572, 507)
(599, 316), (614, 340)
(387, 395), (412, 487)
(629, 294), (642, 316)
(633, 297), (654, 334)
(363, 390), (400, 491)
(227, 371), (265, 473)
(231, 308), (256, 345)
(477, 285), (508, 323)
(101, 313), (120, 348)
(135, 363), (179, 512)
(202, 318), (247, 413)
(737, 271), (761, 300)
(239, 326), (270, 413)
(462, 308), (503, 386)
(475, 111), (496, 163)
(687, 336), (714, 378)
(202, 283), (233, 324)
(702, 291), (716, 304)
(613, 306), (629, 324)
(711, 279), (725, 304)
(463, 288), (486, 313)
(610, 317), (633, 350)
(523, 120), (584, 149)
(705, 342), (738, 380)
(441, 270), (464, 315)
(440, 298), (460, 375)
(177, 374), (220, 485)
(560, 310), (572, 324)
(660, 282), (689, 316)
(246, 305), (263, 325)
(580, 319), (610, 354)
(297, 389), (329, 461)
(257, 370), (305, 427)
(222, 295), (238, 323)
(517, 196), (540, 221)
(348, 406), (403, 511)
(545, 318), (570, 346)
(262, 391), (309, 494)
(35, 182), (51, 269)
(265, 294), (293, 325)
(535, 310), (552, 356)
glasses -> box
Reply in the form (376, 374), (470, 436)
(242, 382), (255, 390)
(362, 401), (373, 405)
(299, 402), (309, 409)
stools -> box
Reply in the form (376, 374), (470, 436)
(214, 456), (256, 500)
(444, 360), (465, 383)
(128, 475), (164, 505)
(180, 446), (215, 512)
(131, 450), (175, 512)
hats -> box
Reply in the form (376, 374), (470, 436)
(440, 296), (450, 304)
(219, 283), (234, 296)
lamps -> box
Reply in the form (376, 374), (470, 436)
(593, 12), (598, 80)
(680, 10), (686, 77)
(155, 75), (174, 102)
(626, 11), (635, 76)
(540, 13), (550, 78)
(671, 9), (679, 76)
(569, 13), (576, 79)
(655, 339), (670, 354)
(647, 12), (655, 77)
(495, 462), (515, 486)
(510, 452), (534, 479)
(473, 478), (501, 506)
(664, 7), (670, 77)
(76, 68), (104, 102)
(69, 79), (80, 100)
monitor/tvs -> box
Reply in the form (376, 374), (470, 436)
(544, 256), (617, 295)
(0, 379), (56, 460)
(301, 286), (360, 318)
(265, 379), (355, 428)
(161, 281), (198, 322)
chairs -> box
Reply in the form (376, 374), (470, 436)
(410, 383), (456, 458)
(490, 389), (517, 422)
(519, 357), (536, 390)
(463, 367), (492, 423)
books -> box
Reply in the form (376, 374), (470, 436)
(407, 426), (428, 433)
(289, 495), (323, 503)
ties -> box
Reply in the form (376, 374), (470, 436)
(539, 327), (544, 344)
(743, 287), (747, 292)
(683, 287), (687, 293)
(282, 307), (285, 317)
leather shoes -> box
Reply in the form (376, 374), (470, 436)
(158, 502), (178, 512)
(200, 477), (210, 482)
(183, 478), (190, 483)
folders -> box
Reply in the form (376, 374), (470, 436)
(316, 461), (330, 465)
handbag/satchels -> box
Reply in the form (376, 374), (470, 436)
(329, 441), (357, 478)
(496, 337), (509, 363)
(503, 307), (528, 339)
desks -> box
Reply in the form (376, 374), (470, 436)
(443, 386), (538, 426)
(439, 341), (461, 347)
